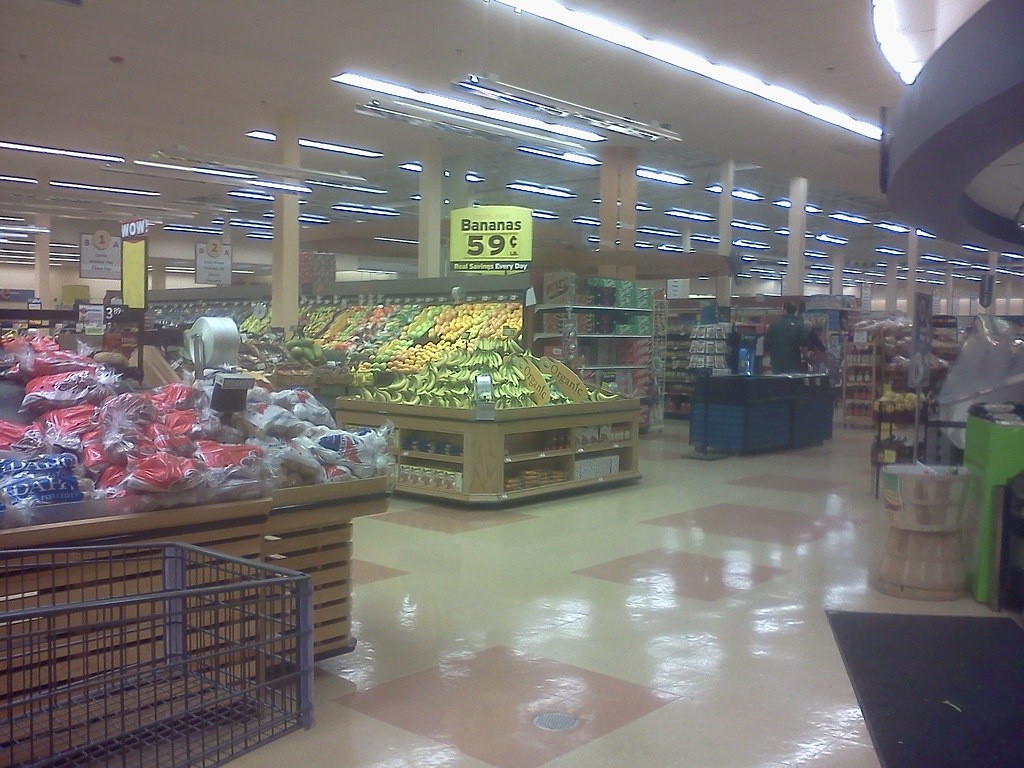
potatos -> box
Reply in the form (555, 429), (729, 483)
(0, 370), (382, 512)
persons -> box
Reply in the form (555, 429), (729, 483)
(761, 301), (828, 376)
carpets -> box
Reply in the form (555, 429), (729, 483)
(824, 608), (1024, 768)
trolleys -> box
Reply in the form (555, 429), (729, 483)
(0, 541), (313, 768)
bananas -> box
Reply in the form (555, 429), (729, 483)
(351, 337), (620, 409)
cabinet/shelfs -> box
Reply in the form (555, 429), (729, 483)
(529, 305), (655, 437)
(871, 401), (925, 501)
(665, 308), (703, 422)
(840, 339), (885, 431)
(335, 393), (642, 509)
(805, 300), (864, 387)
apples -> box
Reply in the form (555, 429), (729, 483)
(357, 301), (522, 374)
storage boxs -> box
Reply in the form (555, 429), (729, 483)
(541, 272), (655, 426)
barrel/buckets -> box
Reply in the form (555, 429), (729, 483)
(867, 464), (978, 601)
(701, 306), (718, 325)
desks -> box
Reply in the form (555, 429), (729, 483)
(0, 474), (396, 768)
